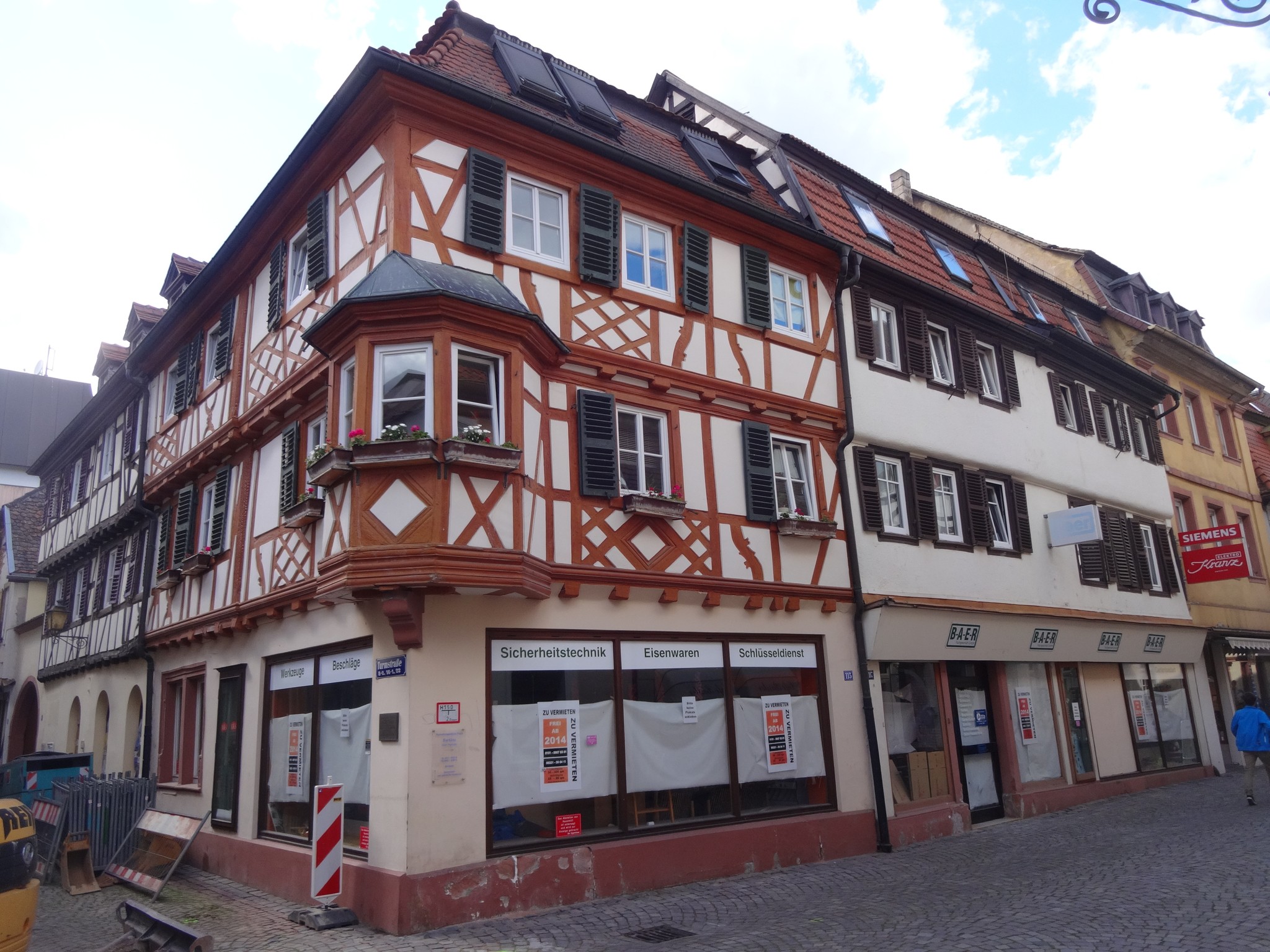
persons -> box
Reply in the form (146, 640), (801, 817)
(1231, 692), (1270, 806)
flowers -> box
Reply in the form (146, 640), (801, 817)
(299, 488), (316, 501)
(304, 439), (343, 467)
(155, 566), (173, 576)
(648, 484), (685, 502)
(780, 508), (836, 524)
(452, 425), (519, 449)
(185, 546), (211, 559)
(348, 423), (430, 446)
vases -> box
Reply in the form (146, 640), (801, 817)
(346, 437), (440, 468)
(623, 494), (687, 520)
(156, 570), (181, 589)
(282, 498), (324, 530)
(777, 518), (838, 539)
(305, 448), (352, 487)
(181, 553), (210, 576)
(442, 439), (523, 472)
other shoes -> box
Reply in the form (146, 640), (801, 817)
(1246, 795), (1258, 805)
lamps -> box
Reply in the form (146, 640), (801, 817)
(47, 598), (88, 649)
